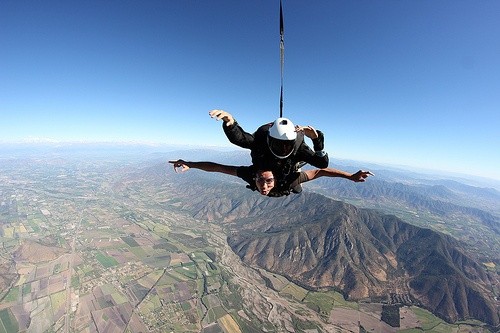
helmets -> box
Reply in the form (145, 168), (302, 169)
(269, 118), (298, 140)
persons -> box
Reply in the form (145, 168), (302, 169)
(209, 109), (329, 169)
(169, 156), (376, 197)
